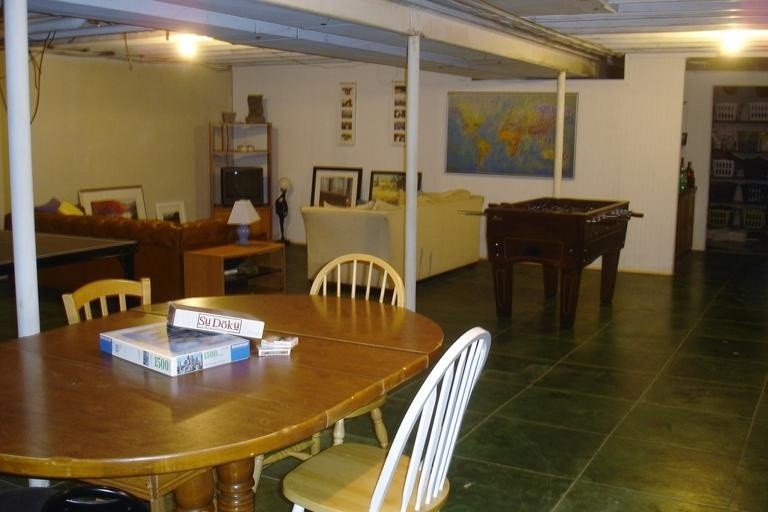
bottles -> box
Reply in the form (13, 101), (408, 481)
(679, 166), (688, 193)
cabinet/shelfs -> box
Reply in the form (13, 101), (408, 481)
(181, 242), (288, 299)
(675, 186), (700, 257)
(207, 120), (274, 240)
(707, 119), (767, 244)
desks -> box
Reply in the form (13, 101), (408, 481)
(2, 291), (447, 510)
(0, 227), (138, 340)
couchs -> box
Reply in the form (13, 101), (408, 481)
(300, 190), (488, 291)
(6, 199), (265, 303)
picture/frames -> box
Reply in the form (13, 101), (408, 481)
(310, 166), (363, 206)
(389, 80), (407, 146)
(369, 169), (423, 207)
(77, 184), (149, 220)
(336, 82), (357, 146)
(153, 201), (188, 224)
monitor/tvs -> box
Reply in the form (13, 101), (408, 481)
(221, 167), (264, 207)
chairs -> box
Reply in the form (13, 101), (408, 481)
(250, 252), (410, 503)
(60, 276), (222, 512)
(282, 326), (492, 511)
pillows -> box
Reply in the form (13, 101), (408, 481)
(55, 201), (85, 218)
(34, 197), (62, 213)
(323, 190), (469, 211)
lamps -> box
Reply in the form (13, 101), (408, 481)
(227, 198), (262, 247)
(273, 178), (293, 247)
